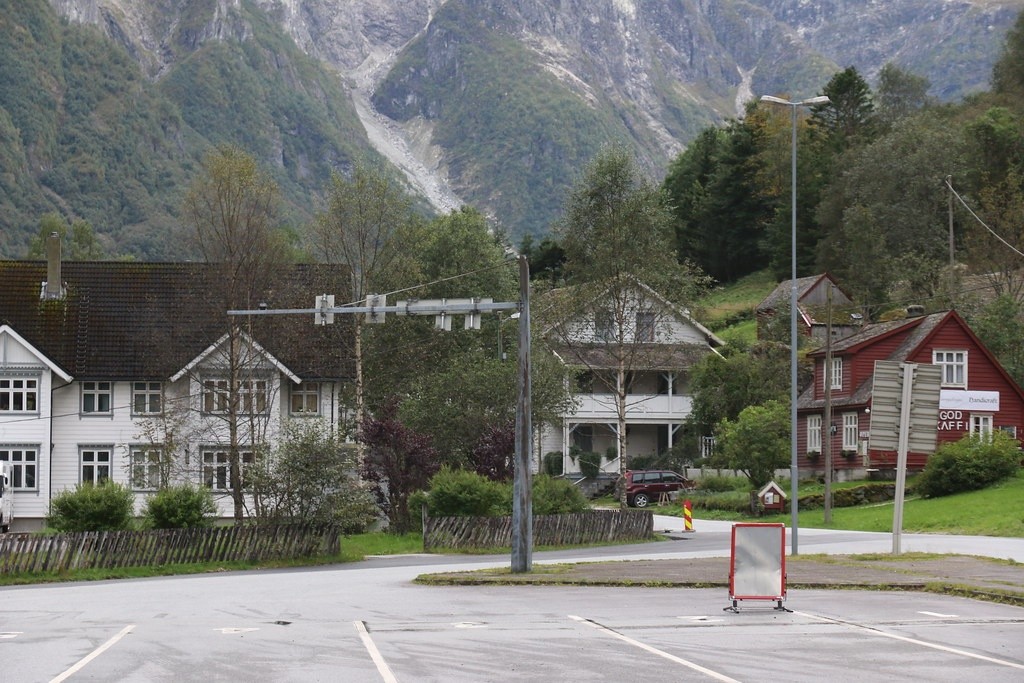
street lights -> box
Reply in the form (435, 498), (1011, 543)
(760, 95), (829, 555)
(498, 313), (520, 362)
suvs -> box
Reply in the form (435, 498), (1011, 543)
(615, 470), (696, 508)
